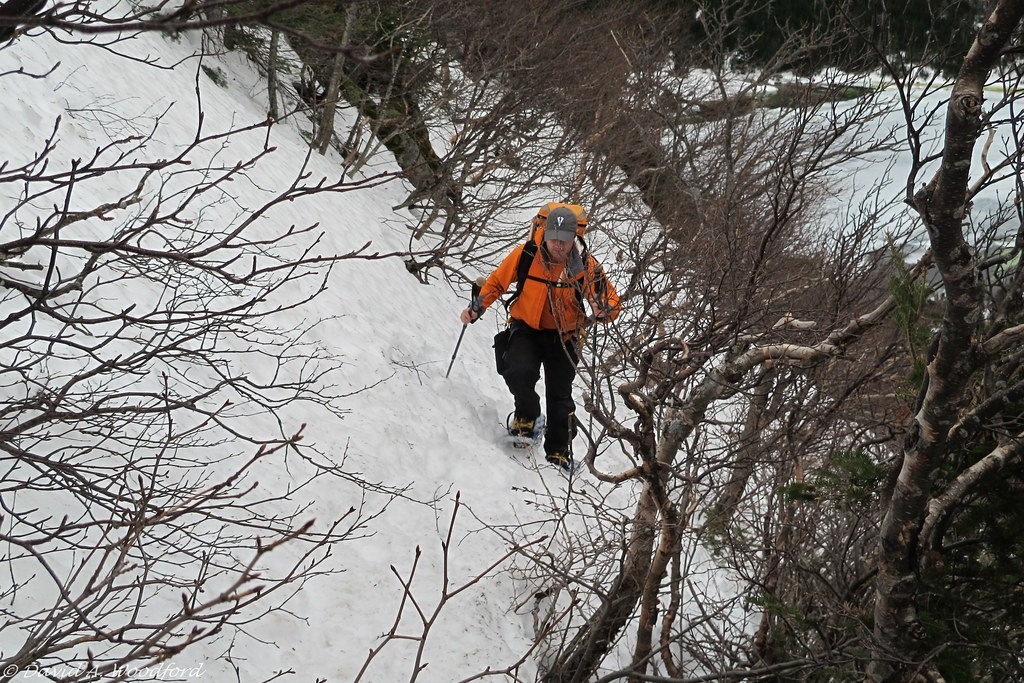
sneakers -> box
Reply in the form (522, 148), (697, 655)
(547, 453), (569, 470)
(512, 414), (536, 448)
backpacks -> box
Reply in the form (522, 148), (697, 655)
(504, 200), (589, 334)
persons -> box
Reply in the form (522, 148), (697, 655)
(460, 206), (621, 468)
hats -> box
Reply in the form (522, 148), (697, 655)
(544, 206), (576, 242)
